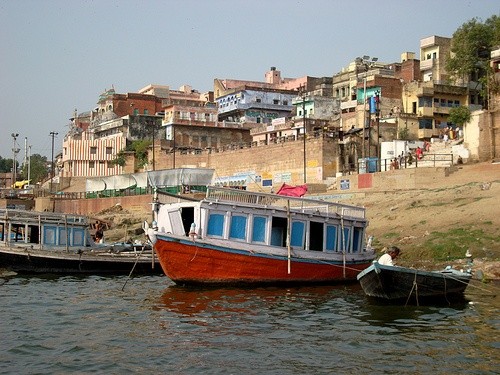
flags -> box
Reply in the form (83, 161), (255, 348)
(277, 183), (309, 197)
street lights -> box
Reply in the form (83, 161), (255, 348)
(10, 132), (19, 187)
(362, 56), (380, 167)
(50, 131), (60, 196)
(297, 89), (308, 181)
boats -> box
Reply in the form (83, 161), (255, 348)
(140, 165), (375, 284)
(0, 208), (164, 272)
(356, 259), (474, 303)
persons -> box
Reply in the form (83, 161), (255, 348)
(440, 126), (459, 140)
(391, 140), (429, 169)
(95, 221), (104, 243)
(378, 246), (401, 266)
